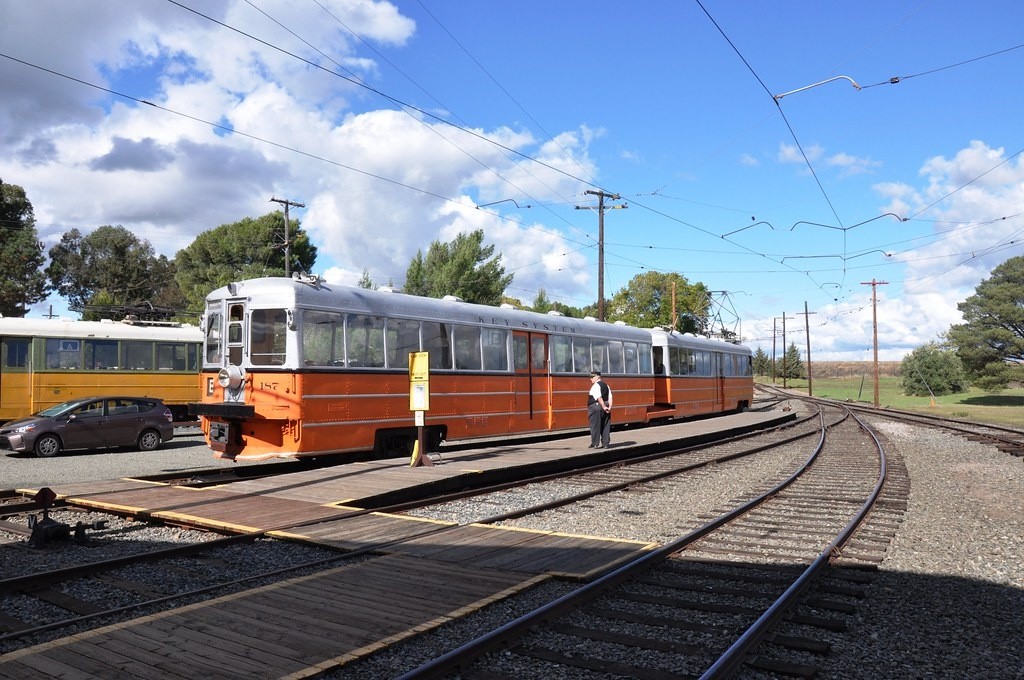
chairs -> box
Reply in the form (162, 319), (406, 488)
(268, 356), (486, 369)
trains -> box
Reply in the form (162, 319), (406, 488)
(199, 276), (754, 463)
(0, 314), (203, 424)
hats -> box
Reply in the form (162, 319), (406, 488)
(589, 371), (601, 377)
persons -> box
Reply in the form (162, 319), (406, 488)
(587, 371), (614, 449)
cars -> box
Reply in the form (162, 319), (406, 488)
(0, 395), (174, 459)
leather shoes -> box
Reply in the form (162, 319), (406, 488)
(586, 444), (597, 448)
(600, 445), (609, 448)
